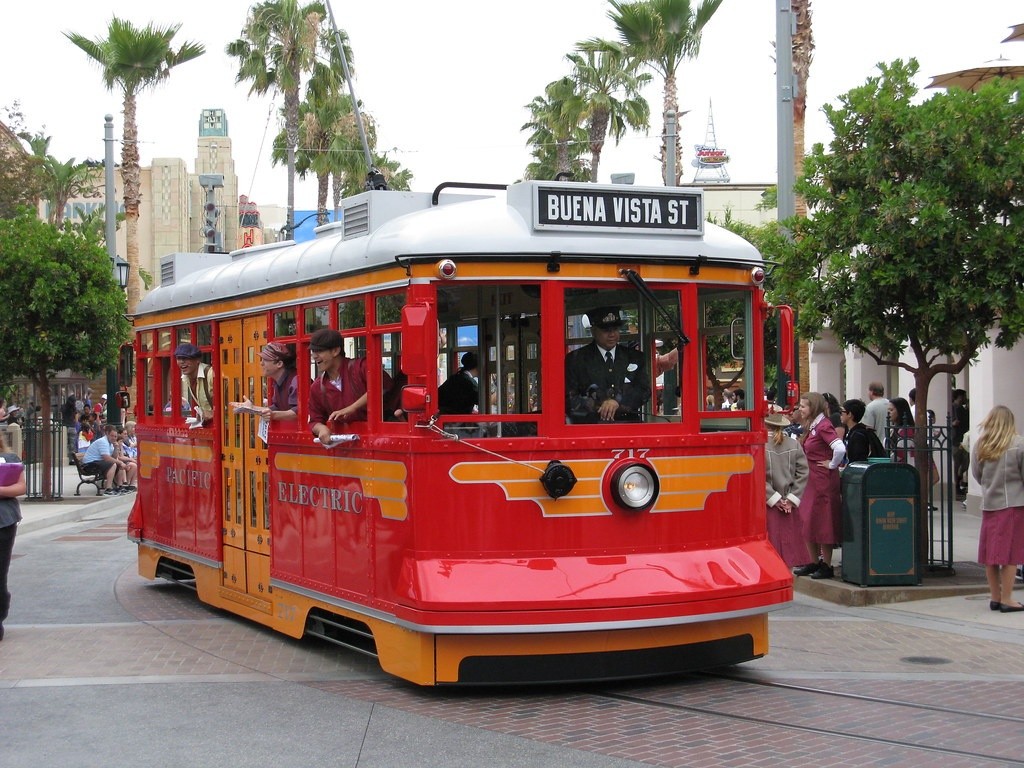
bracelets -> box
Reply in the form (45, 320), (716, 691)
(120, 462), (123, 465)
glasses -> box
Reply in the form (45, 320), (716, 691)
(839, 410), (847, 415)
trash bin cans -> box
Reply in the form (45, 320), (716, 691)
(841, 460), (920, 586)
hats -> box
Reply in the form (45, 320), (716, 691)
(257, 342), (296, 362)
(764, 404), (790, 427)
(88, 388), (93, 393)
(7, 405), (19, 414)
(307, 329), (342, 349)
(102, 394), (107, 400)
(461, 353), (478, 365)
(584, 307), (627, 327)
(175, 343), (201, 359)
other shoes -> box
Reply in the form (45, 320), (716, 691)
(117, 482), (137, 494)
(956, 488), (968, 495)
(990, 601), (1024, 613)
(793, 559), (823, 576)
(958, 468), (963, 481)
(928, 503), (938, 511)
(104, 489), (119, 497)
(811, 562), (834, 579)
(69, 460), (76, 465)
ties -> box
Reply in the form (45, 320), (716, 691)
(605, 352), (613, 369)
(276, 384), (286, 411)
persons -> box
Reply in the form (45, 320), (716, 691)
(228, 342), (297, 422)
(306, 329), (407, 443)
(706, 388), (745, 410)
(858, 383), (892, 445)
(565, 306), (651, 424)
(794, 392), (846, 580)
(821, 393), (848, 444)
(883, 398), (915, 467)
(908, 388), (940, 511)
(0, 431), (37, 641)
(840, 399), (867, 460)
(529, 376), (538, 412)
(947, 389), (969, 494)
(0, 388), (138, 495)
(764, 386), (803, 440)
(174, 343), (214, 426)
(437, 349), (482, 414)
(972, 405), (1024, 613)
(764, 404), (814, 567)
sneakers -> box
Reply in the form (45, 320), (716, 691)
(0, 622), (4, 641)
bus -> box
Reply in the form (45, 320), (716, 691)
(116, 179), (799, 688)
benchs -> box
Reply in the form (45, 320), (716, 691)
(71, 451), (107, 496)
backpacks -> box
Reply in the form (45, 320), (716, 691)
(850, 428), (887, 457)
